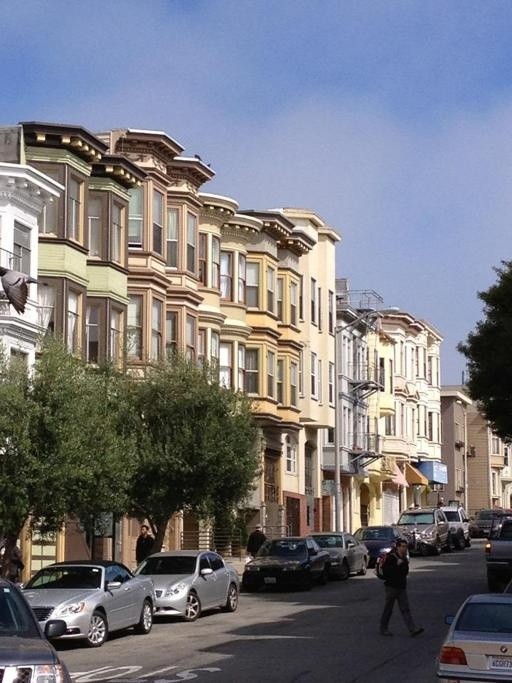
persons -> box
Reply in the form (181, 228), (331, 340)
(379, 539), (424, 637)
(245, 524), (267, 559)
(0, 532), (22, 584)
(136, 525), (154, 568)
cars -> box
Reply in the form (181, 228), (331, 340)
(427, 589), (512, 682)
(19, 558), (156, 650)
(242, 498), (512, 589)
(0, 575), (73, 683)
(130, 548), (240, 623)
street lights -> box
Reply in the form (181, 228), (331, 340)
(332, 304), (400, 532)
(455, 399), (473, 514)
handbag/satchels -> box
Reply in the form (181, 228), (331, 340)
(375, 552), (398, 579)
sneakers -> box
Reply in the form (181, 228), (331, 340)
(379, 630), (392, 635)
(411, 627), (423, 637)
